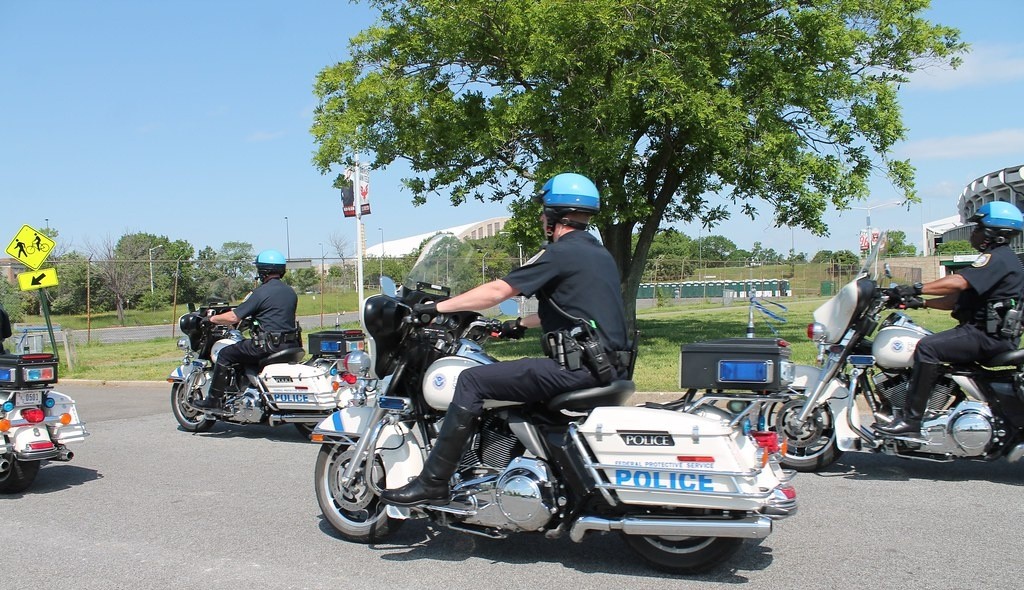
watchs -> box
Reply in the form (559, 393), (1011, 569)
(913, 281), (922, 294)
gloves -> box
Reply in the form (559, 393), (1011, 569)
(885, 285), (923, 310)
(497, 317), (527, 340)
(200, 315), (217, 329)
(411, 297), (441, 325)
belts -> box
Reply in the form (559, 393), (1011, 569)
(616, 350), (631, 367)
(268, 333), (296, 341)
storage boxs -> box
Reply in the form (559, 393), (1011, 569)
(678, 337), (796, 391)
(0, 354), (59, 383)
(307, 330), (366, 355)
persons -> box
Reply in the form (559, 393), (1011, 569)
(193, 248), (298, 413)
(871, 200), (1024, 441)
(379, 174), (633, 508)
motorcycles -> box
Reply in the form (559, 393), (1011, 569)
(165, 302), (378, 442)
(0, 306), (91, 496)
(309, 277), (800, 573)
(765, 232), (1023, 471)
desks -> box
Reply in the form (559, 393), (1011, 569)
(14, 328), (78, 371)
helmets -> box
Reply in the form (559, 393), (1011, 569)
(974, 201), (1024, 231)
(252, 250), (287, 278)
(539, 173), (599, 209)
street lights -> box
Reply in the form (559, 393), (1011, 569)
(285, 216), (290, 261)
(148, 244), (164, 298)
(377, 227), (384, 260)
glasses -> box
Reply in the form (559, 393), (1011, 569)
(539, 210), (546, 216)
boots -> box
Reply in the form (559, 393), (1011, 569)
(195, 362), (233, 407)
(870, 363), (943, 437)
(380, 402), (483, 507)
